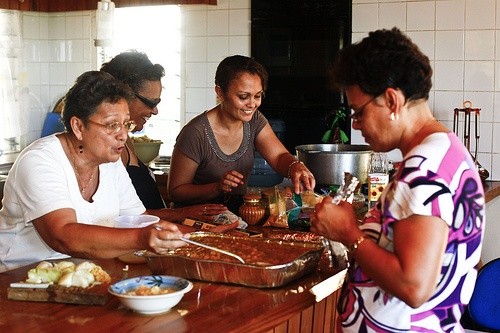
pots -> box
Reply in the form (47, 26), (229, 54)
(296, 143), (373, 186)
(247, 158), (284, 187)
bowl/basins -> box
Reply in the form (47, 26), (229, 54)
(106, 275), (193, 315)
(132, 139), (163, 168)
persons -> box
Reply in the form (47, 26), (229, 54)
(100, 49), (164, 210)
(167, 55), (316, 209)
(311, 26), (486, 333)
(0, 70), (190, 274)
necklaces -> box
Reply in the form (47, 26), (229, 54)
(70, 151), (96, 193)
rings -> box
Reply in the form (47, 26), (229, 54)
(313, 210), (316, 213)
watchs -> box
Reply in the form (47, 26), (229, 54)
(348, 235), (366, 257)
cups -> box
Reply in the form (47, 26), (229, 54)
(113, 214), (160, 264)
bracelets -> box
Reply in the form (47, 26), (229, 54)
(288, 160), (304, 178)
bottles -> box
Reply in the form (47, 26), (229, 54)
(238, 195), (265, 226)
(367, 152), (389, 211)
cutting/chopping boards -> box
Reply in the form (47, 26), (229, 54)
(6, 269), (128, 307)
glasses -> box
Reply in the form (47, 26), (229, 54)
(76, 116), (136, 135)
(102, 62), (162, 109)
(349, 87), (398, 120)
